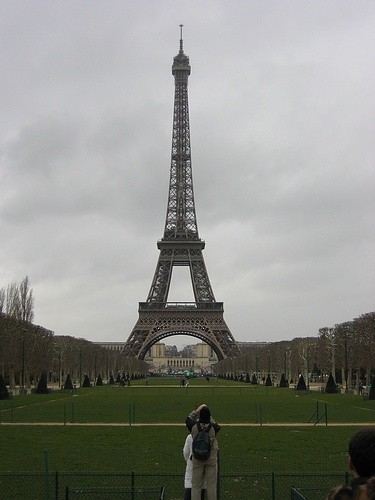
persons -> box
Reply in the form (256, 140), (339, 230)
(333, 426), (375, 500)
(122, 375), (130, 386)
(182, 378), (190, 387)
(182, 404), (221, 500)
(206, 371), (210, 383)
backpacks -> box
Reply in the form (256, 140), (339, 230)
(192, 421), (213, 461)
